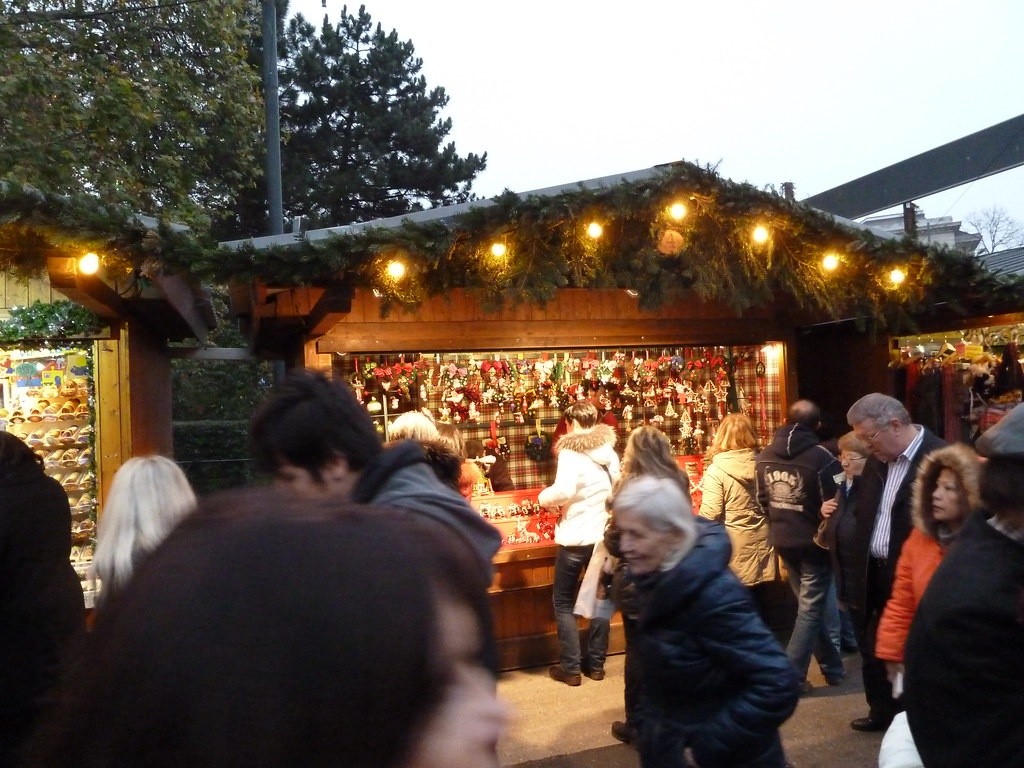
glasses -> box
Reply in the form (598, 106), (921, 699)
(838, 454), (866, 462)
(859, 419), (890, 443)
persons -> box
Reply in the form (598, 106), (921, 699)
(602, 424), (690, 751)
(613, 475), (801, 768)
(537, 401), (621, 688)
(754, 343), (1024, 767)
(697, 412), (789, 639)
(0, 366), (519, 768)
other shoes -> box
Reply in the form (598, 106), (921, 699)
(612, 720), (641, 748)
(581, 664), (604, 680)
(828, 669), (847, 684)
(550, 666), (580, 686)
(800, 680), (812, 694)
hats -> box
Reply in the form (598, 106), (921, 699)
(975, 402), (1023, 461)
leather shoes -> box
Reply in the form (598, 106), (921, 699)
(851, 716), (885, 731)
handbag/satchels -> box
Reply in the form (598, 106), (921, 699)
(814, 488), (840, 550)
(573, 539), (621, 621)
